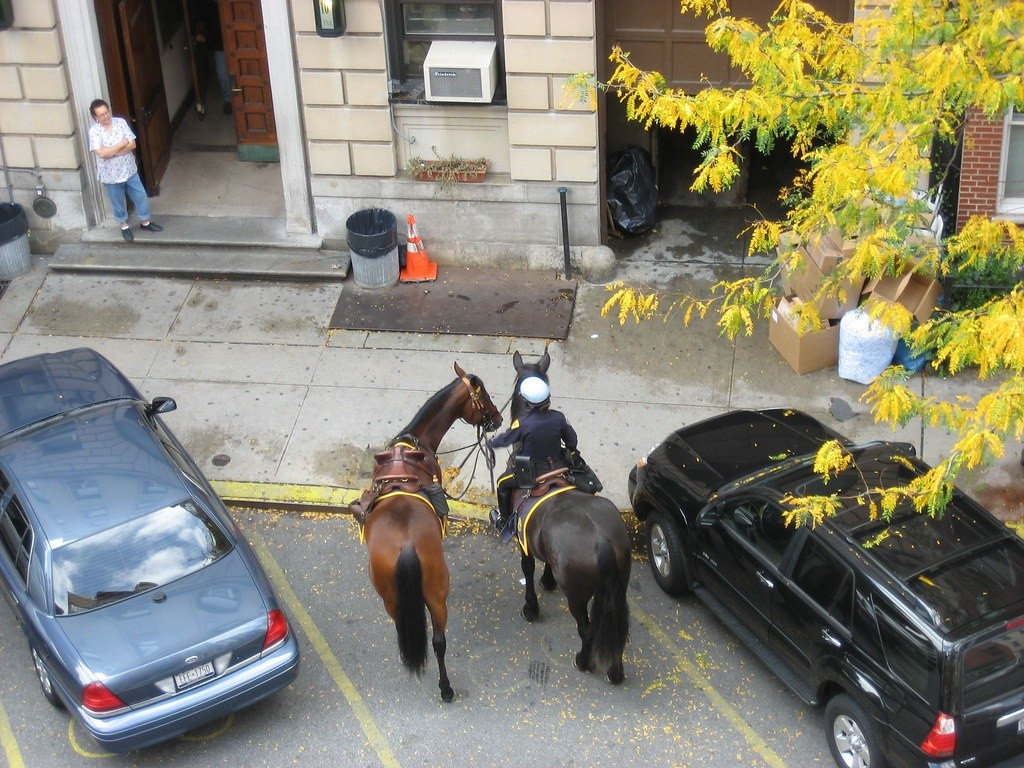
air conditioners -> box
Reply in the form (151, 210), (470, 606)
(422, 41), (499, 103)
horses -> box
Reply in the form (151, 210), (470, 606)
(364, 350), (633, 703)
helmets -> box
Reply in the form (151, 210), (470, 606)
(516, 373), (550, 404)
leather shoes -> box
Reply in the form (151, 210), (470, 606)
(140, 222), (163, 232)
(121, 226), (133, 241)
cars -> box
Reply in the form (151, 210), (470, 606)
(0, 348), (300, 753)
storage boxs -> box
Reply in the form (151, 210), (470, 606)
(768, 189), (945, 375)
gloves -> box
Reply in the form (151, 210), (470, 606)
(486, 438), (494, 449)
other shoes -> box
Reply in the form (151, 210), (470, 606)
(222, 102), (232, 114)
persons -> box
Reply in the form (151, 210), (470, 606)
(88, 99), (163, 241)
(488, 377), (578, 530)
(195, 20), (232, 114)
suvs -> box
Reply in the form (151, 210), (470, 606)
(628, 407), (1024, 768)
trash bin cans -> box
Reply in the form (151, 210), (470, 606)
(0, 203), (31, 275)
(346, 208), (401, 288)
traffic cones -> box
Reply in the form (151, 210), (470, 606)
(399, 213), (438, 283)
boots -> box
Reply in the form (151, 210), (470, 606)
(489, 488), (512, 530)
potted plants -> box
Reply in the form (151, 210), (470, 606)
(410, 148), (493, 181)
